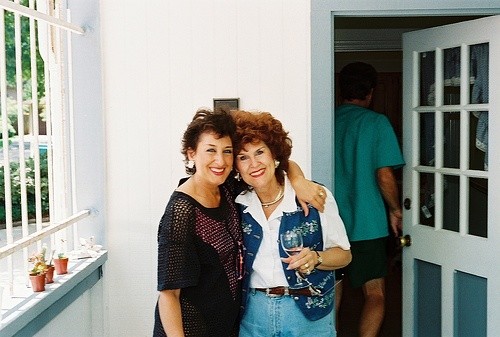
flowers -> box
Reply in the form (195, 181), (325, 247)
(29, 239), (67, 274)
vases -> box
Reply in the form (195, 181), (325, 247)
(53, 257), (69, 275)
(44, 265), (55, 284)
(28, 271), (47, 292)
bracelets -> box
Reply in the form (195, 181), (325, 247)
(311, 249), (323, 268)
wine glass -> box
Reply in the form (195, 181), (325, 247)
(280, 230), (312, 290)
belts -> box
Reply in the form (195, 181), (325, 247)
(254, 287), (311, 297)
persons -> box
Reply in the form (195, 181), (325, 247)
(153, 105), (327, 337)
(228, 109), (352, 336)
(335, 61), (406, 337)
(261, 187), (284, 208)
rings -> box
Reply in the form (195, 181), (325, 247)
(306, 269), (311, 275)
(319, 192), (324, 197)
(305, 263), (310, 268)
